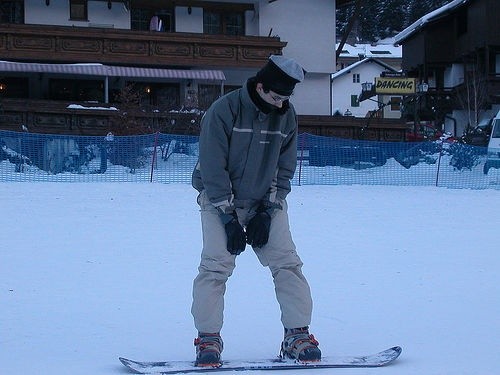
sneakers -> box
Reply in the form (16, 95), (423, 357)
(279, 327), (322, 362)
(194, 331), (224, 366)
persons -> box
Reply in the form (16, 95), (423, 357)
(333, 109), (340, 116)
(192, 57), (321, 365)
(408, 62), (434, 79)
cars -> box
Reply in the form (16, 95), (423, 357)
(466, 118), (493, 145)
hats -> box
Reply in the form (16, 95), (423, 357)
(257, 54), (307, 93)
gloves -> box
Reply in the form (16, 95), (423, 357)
(245, 209), (272, 248)
(222, 213), (246, 256)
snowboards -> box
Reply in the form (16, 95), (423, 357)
(119, 345), (402, 375)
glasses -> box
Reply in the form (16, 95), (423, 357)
(268, 91), (290, 103)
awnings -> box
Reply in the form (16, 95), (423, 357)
(0, 61), (226, 96)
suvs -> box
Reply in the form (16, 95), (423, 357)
(404, 123), (458, 143)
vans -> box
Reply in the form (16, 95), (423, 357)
(486, 110), (500, 164)
